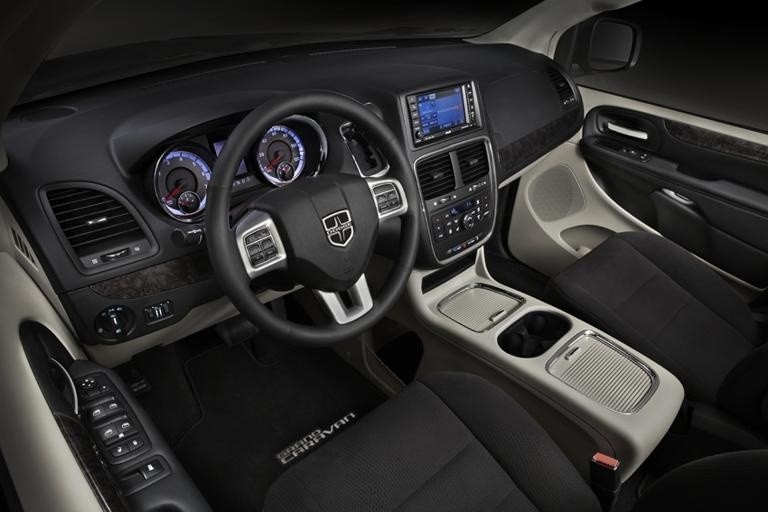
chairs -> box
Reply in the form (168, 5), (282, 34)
(548, 230), (768, 448)
(260, 370), (768, 512)
